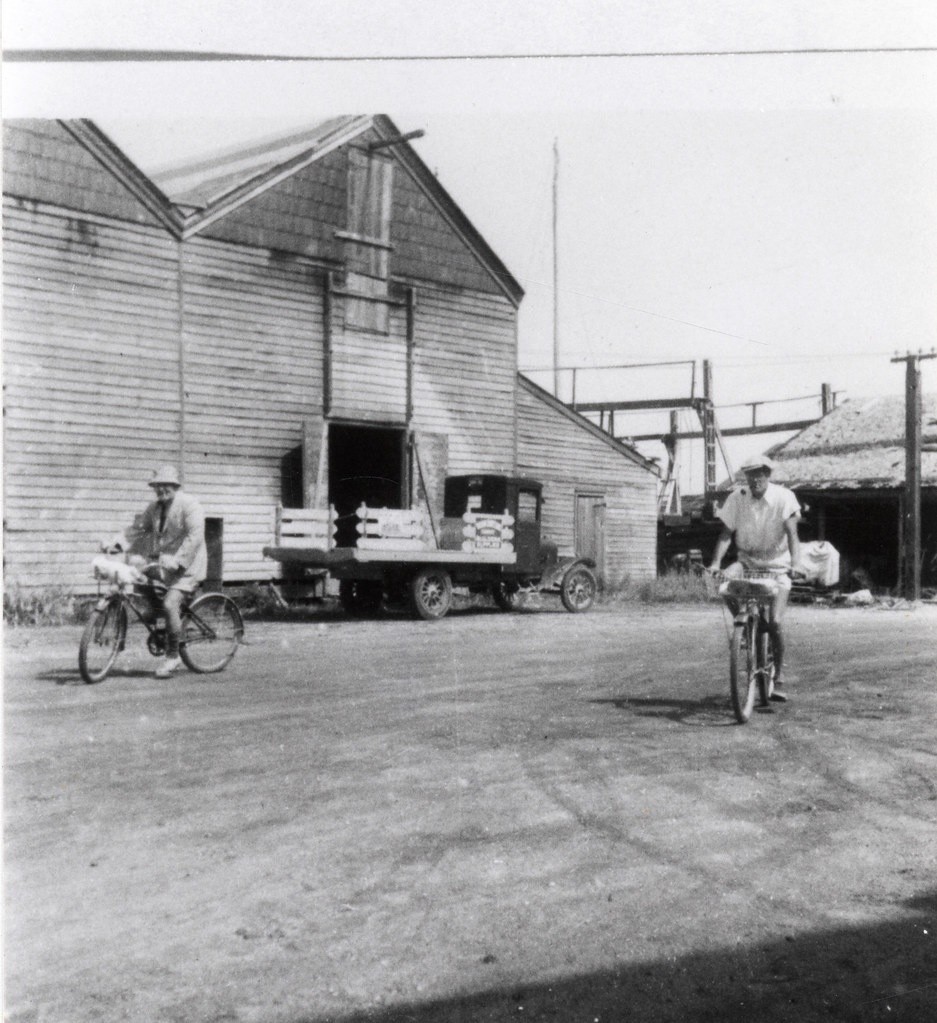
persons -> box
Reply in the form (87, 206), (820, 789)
(102, 465), (208, 678)
(706, 455), (801, 684)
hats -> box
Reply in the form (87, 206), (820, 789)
(740, 455), (776, 474)
(149, 465), (182, 490)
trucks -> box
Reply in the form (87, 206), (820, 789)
(262, 475), (599, 618)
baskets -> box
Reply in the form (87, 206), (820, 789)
(717, 570), (777, 598)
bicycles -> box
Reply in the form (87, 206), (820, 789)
(78, 539), (245, 686)
(710, 565), (781, 722)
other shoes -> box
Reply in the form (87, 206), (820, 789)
(155, 656), (179, 677)
(770, 689), (787, 702)
